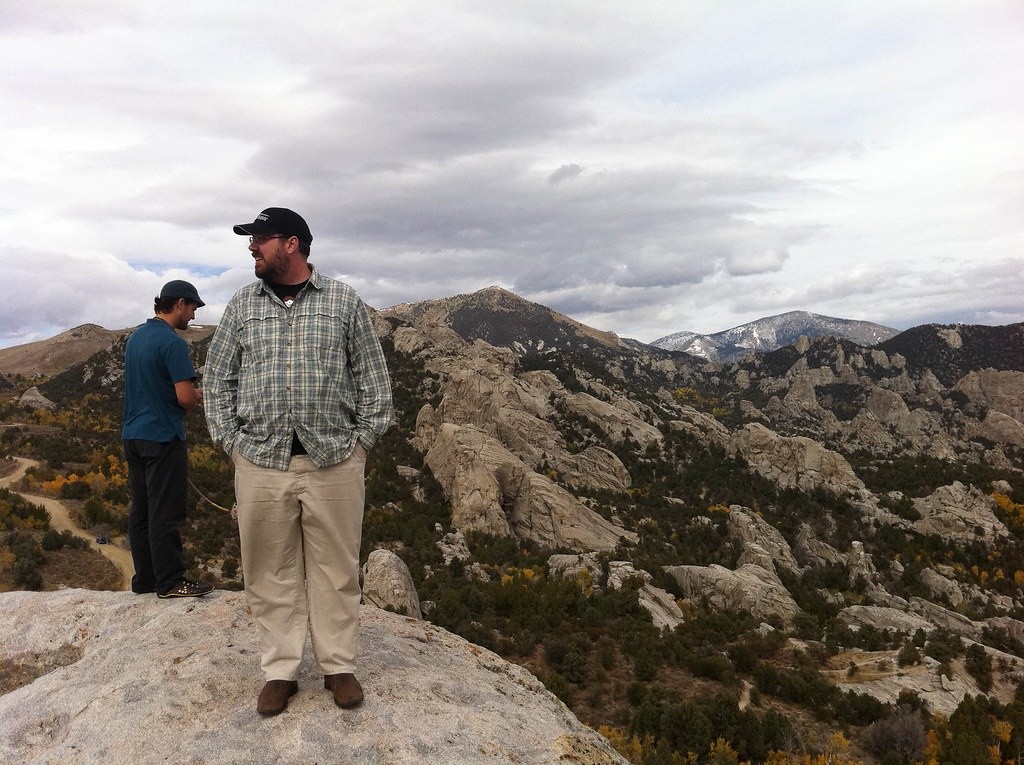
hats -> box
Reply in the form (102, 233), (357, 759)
(233, 207), (313, 244)
(160, 280), (205, 305)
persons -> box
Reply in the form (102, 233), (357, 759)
(119, 280), (216, 598)
(203, 208), (391, 716)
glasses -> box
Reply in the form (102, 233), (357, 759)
(249, 237), (290, 244)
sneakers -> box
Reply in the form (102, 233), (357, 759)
(257, 680), (298, 715)
(324, 673), (364, 707)
(157, 579), (215, 598)
